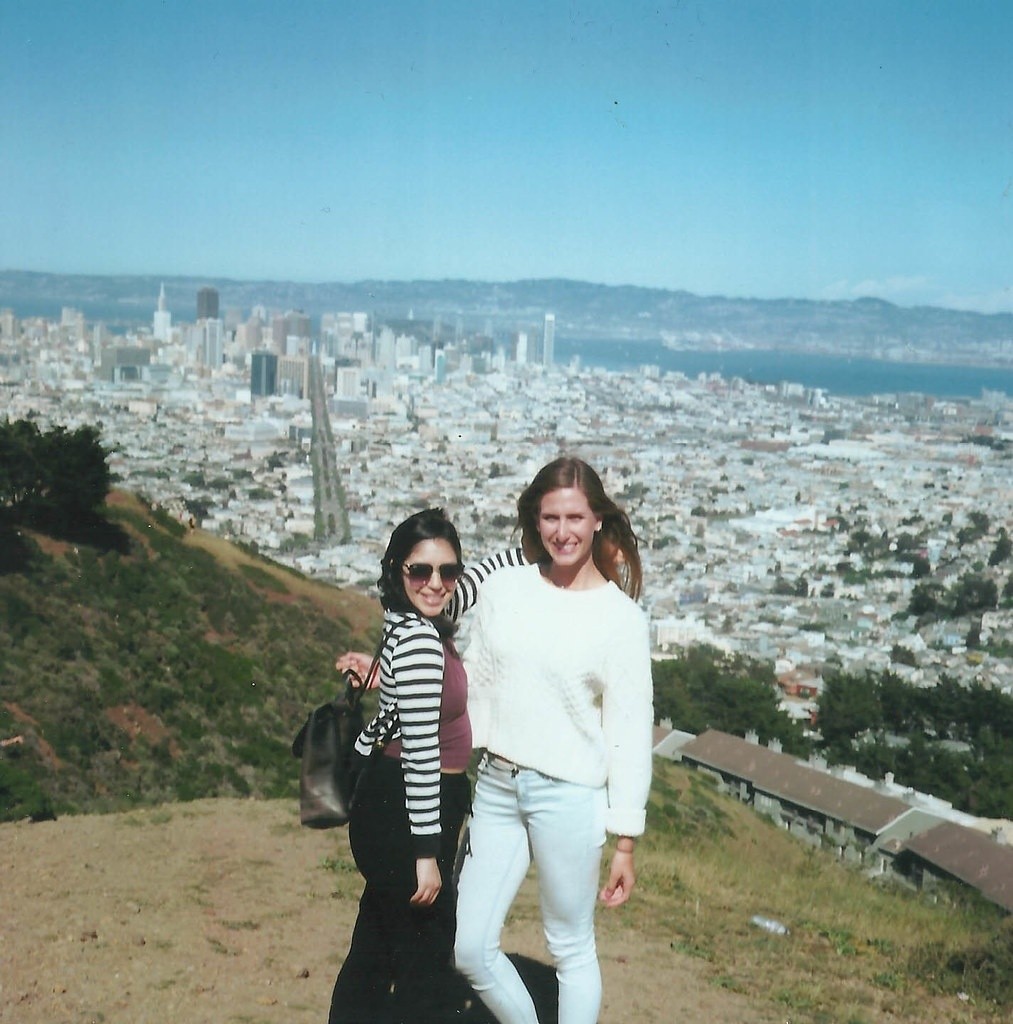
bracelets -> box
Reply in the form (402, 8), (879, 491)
(617, 847), (634, 853)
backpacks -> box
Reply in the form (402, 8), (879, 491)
(292, 616), (427, 830)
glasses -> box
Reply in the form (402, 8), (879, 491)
(403, 562), (466, 589)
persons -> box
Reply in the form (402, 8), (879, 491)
(328, 508), (474, 1024)
(335, 457), (654, 1024)
